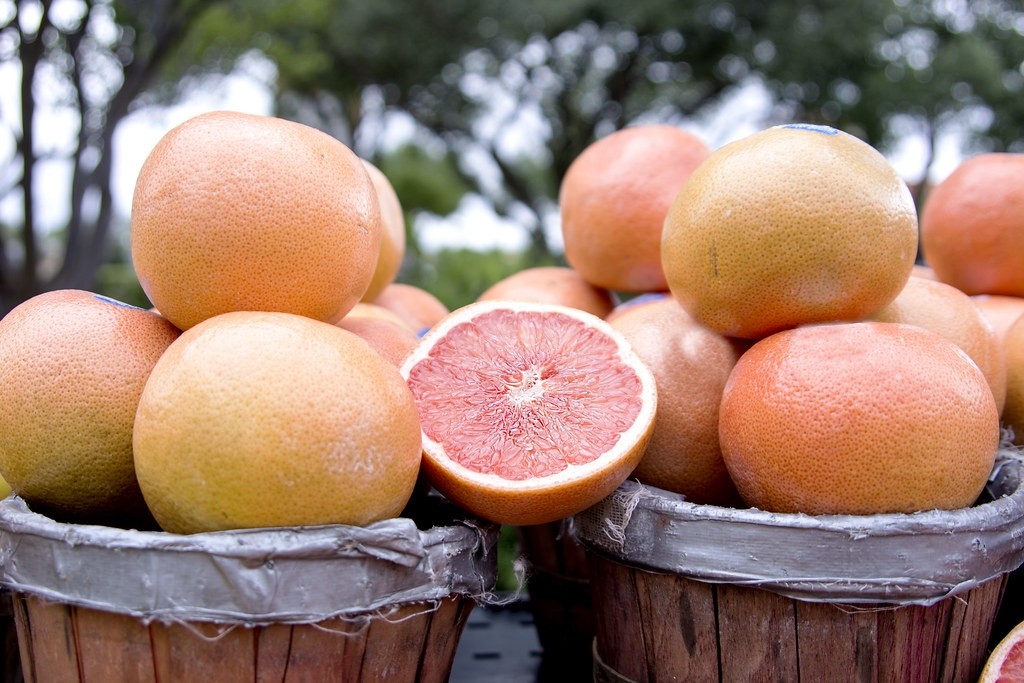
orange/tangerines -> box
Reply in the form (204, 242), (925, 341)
(978, 619), (1024, 683)
(559, 121), (1024, 517)
(0, 109), (659, 532)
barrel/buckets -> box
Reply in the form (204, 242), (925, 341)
(0, 493), (499, 683)
(571, 444), (1024, 683)
